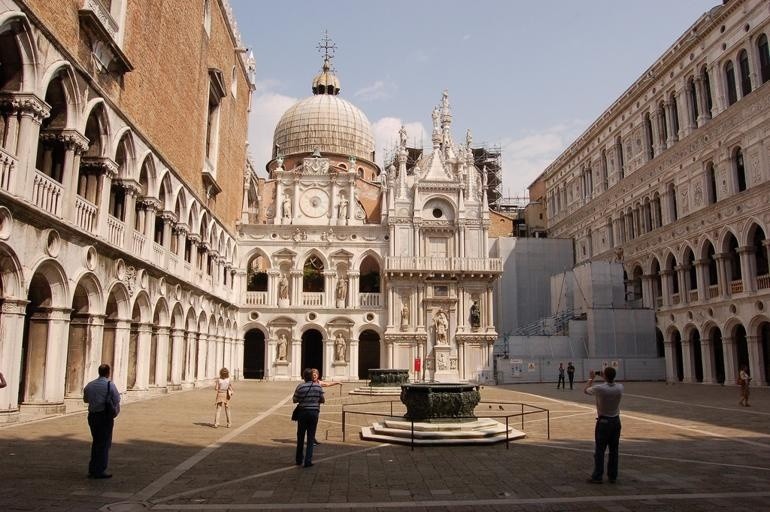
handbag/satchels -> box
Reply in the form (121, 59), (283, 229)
(736, 378), (745, 385)
(292, 405), (302, 420)
(106, 395), (120, 418)
(227, 384), (233, 400)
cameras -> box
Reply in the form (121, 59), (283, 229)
(594, 370), (600, 375)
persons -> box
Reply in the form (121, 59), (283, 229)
(431, 105), (440, 133)
(277, 334), (287, 360)
(311, 368), (343, 446)
(283, 194), (292, 218)
(292, 368), (325, 468)
(279, 273), (288, 300)
(83, 363), (120, 479)
(399, 125), (407, 147)
(557, 363), (565, 389)
(583, 366), (624, 485)
(436, 313), (448, 344)
(337, 274), (347, 300)
(483, 165), (487, 181)
(465, 127), (472, 144)
(0, 372), (8, 389)
(336, 332), (346, 361)
(566, 362), (575, 390)
(471, 300), (480, 327)
(380, 167), (388, 185)
(339, 194), (349, 219)
(401, 303), (409, 325)
(212, 367), (231, 428)
(738, 365), (752, 407)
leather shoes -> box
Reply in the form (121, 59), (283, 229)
(94, 473), (112, 478)
